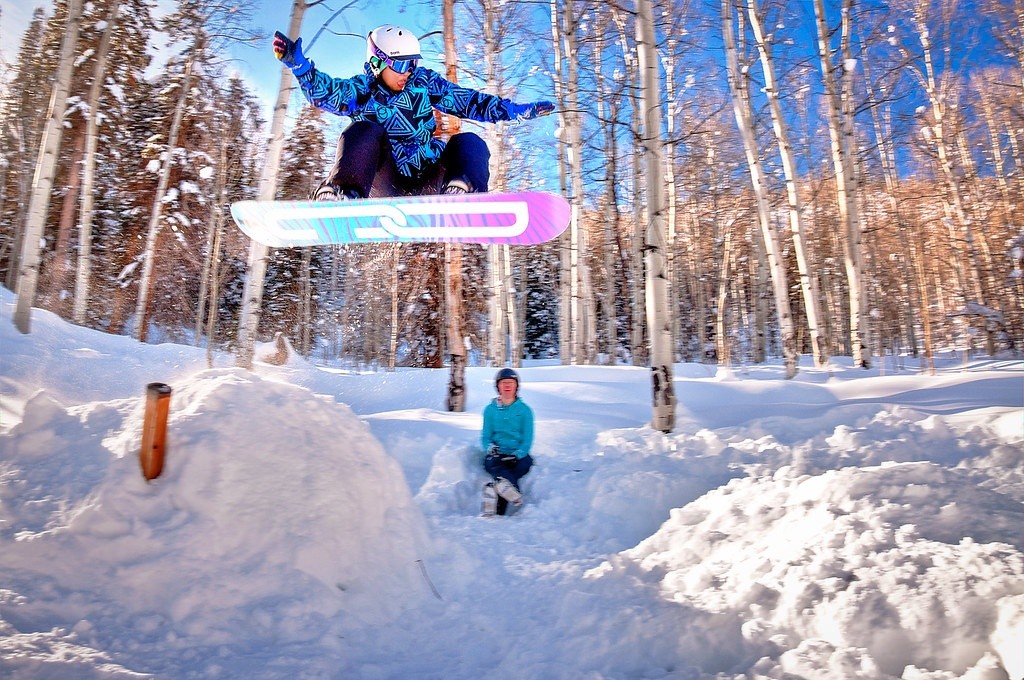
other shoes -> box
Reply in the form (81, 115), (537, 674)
(446, 183), (470, 197)
(317, 185), (361, 202)
(482, 482), (497, 517)
(493, 476), (524, 508)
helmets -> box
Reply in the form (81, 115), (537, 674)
(366, 26), (423, 75)
(496, 368), (520, 385)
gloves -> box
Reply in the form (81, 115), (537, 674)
(509, 101), (554, 122)
(496, 453), (519, 470)
(272, 30), (311, 77)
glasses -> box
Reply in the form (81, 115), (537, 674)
(367, 31), (419, 74)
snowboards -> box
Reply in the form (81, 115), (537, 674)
(230, 192), (572, 248)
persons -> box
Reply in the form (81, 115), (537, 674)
(481, 368), (534, 516)
(274, 22), (555, 200)
